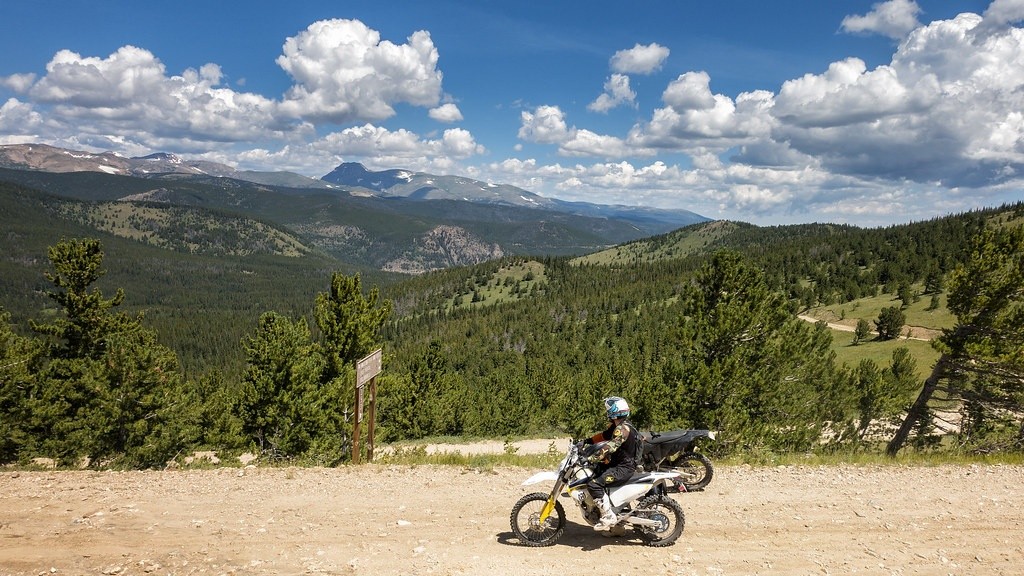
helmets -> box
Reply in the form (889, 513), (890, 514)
(604, 396), (630, 420)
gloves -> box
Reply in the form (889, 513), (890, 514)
(576, 440), (586, 448)
(580, 456), (589, 465)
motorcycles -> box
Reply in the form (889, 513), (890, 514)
(510, 438), (685, 548)
(635, 429), (716, 493)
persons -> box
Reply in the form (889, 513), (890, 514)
(576, 396), (640, 532)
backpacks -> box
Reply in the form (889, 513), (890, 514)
(625, 423), (646, 465)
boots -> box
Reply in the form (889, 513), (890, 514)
(592, 493), (617, 530)
(602, 523), (626, 537)
